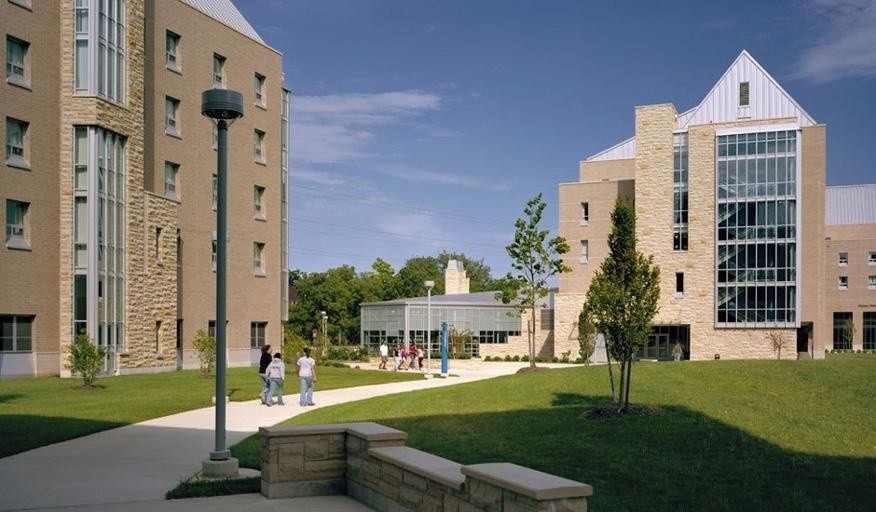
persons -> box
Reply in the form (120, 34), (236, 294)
(672, 341), (683, 361)
(296, 347), (318, 407)
(378, 341), (389, 369)
(416, 345), (425, 370)
(258, 344), (273, 404)
(408, 342), (418, 370)
(398, 344), (408, 370)
(264, 352), (287, 407)
(391, 345), (400, 371)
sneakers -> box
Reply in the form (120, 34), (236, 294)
(258, 393), (284, 406)
(301, 402), (315, 406)
